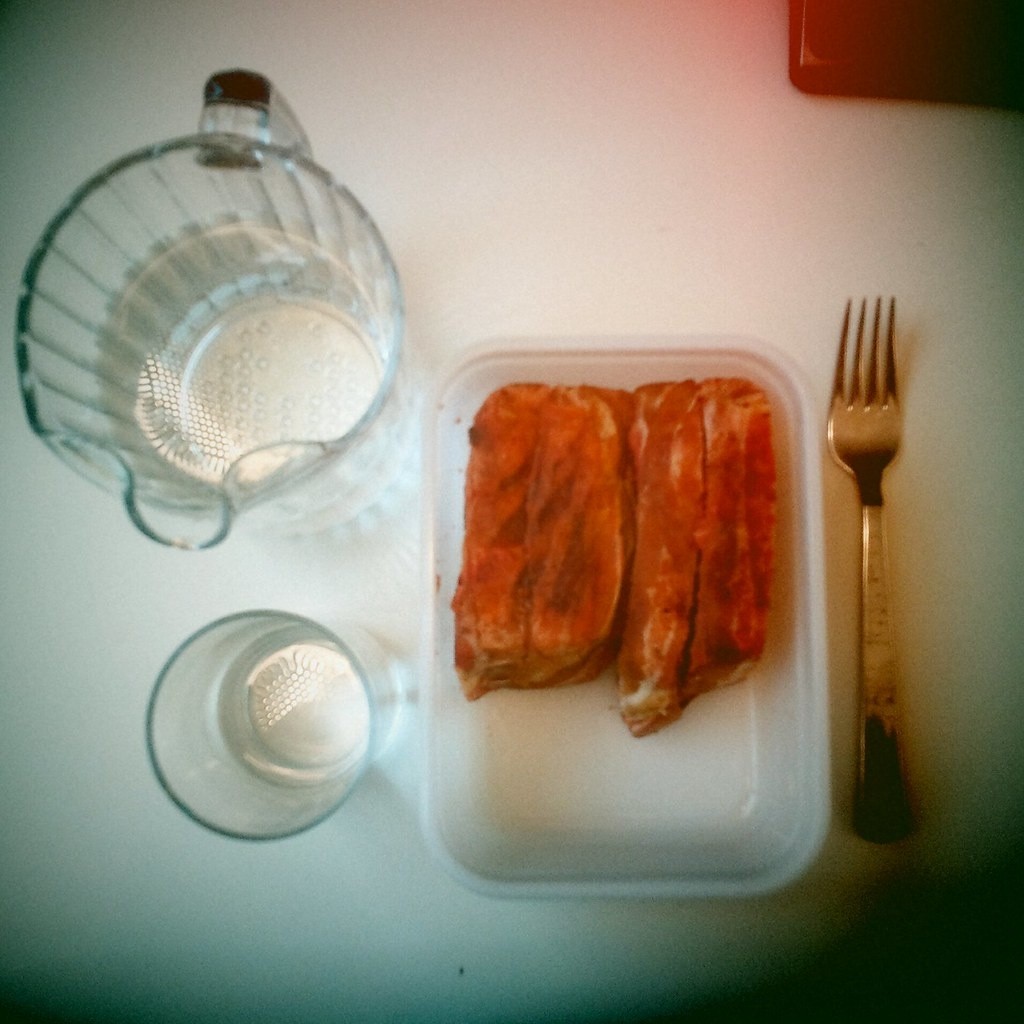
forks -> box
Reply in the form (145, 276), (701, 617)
(826, 293), (919, 844)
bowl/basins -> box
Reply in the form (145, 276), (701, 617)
(404, 330), (825, 907)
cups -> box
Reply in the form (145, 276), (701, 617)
(14, 66), (405, 551)
(143, 608), (412, 844)
